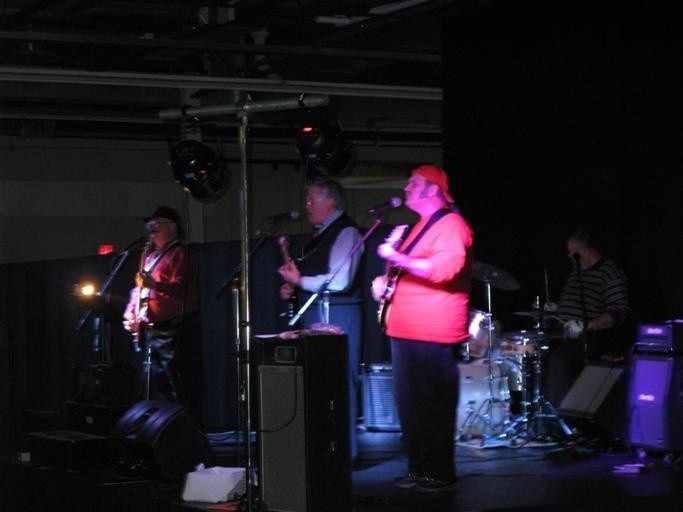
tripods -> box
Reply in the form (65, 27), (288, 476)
(457, 281), (575, 445)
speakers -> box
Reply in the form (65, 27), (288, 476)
(362, 361), (406, 432)
(555, 359), (624, 429)
(109, 398), (216, 489)
(27, 430), (109, 470)
(623, 355), (683, 455)
(255, 332), (350, 512)
(63, 362), (139, 433)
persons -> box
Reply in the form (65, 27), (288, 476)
(277, 176), (369, 469)
(120, 203), (196, 406)
(537, 228), (633, 372)
(369, 165), (476, 492)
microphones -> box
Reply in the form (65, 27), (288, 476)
(264, 210), (300, 226)
(366, 198), (402, 214)
(121, 237), (149, 251)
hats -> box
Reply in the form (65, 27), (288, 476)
(410, 163), (456, 204)
(141, 205), (185, 241)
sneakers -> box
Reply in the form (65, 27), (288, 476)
(393, 472), (458, 493)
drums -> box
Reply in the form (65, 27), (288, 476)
(499, 331), (539, 356)
(457, 361), (510, 438)
(461, 308), (503, 359)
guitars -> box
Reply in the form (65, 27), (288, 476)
(277, 235), (299, 321)
(130, 234), (155, 353)
(377, 225), (410, 327)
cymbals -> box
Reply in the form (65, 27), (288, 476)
(472, 264), (521, 290)
(508, 328), (568, 340)
(515, 309), (564, 317)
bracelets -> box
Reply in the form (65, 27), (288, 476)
(297, 274), (301, 286)
